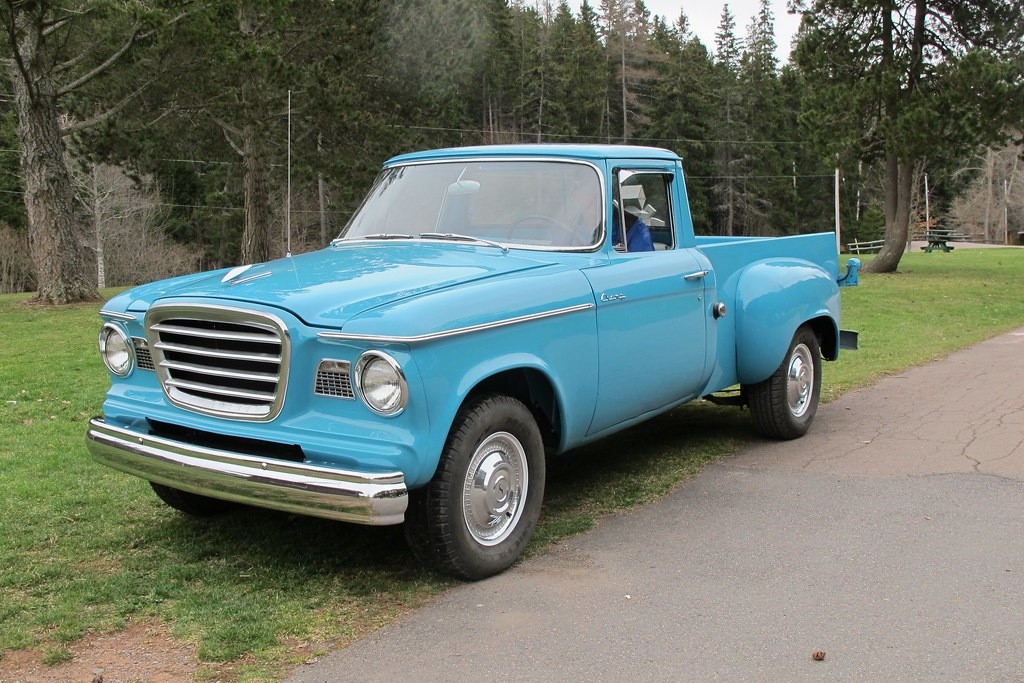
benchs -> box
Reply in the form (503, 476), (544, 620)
(921, 246), (954, 253)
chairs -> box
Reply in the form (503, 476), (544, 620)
(648, 227), (673, 249)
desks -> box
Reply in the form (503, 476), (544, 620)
(925, 240), (949, 254)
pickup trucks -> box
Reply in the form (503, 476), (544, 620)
(85, 144), (860, 581)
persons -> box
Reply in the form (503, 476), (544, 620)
(561, 165), (656, 252)
